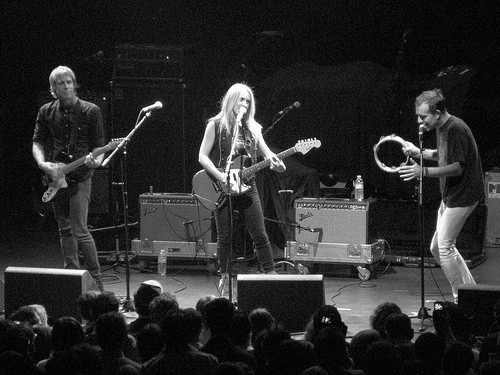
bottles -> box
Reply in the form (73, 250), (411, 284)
(158, 249), (167, 275)
(355, 175), (364, 201)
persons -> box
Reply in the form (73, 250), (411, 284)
(198, 84), (287, 306)
(33, 66), (105, 292)
(1, 281), (500, 375)
(399, 88), (487, 305)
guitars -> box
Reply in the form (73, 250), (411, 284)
(34, 137), (130, 202)
(192, 136), (323, 210)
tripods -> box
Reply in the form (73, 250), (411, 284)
(100, 110), (156, 314)
(404, 127), (434, 333)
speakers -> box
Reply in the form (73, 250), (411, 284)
(293, 199), (371, 243)
(236, 273), (327, 336)
(486, 172), (500, 249)
(457, 283), (500, 314)
(4, 267), (100, 326)
(107, 81), (187, 225)
(139, 194), (218, 242)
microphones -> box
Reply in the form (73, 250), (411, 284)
(142, 100), (163, 112)
(420, 124), (425, 135)
(277, 101), (301, 115)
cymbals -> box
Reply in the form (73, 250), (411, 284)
(373, 132), (411, 173)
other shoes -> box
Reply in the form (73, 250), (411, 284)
(220, 274), (238, 302)
